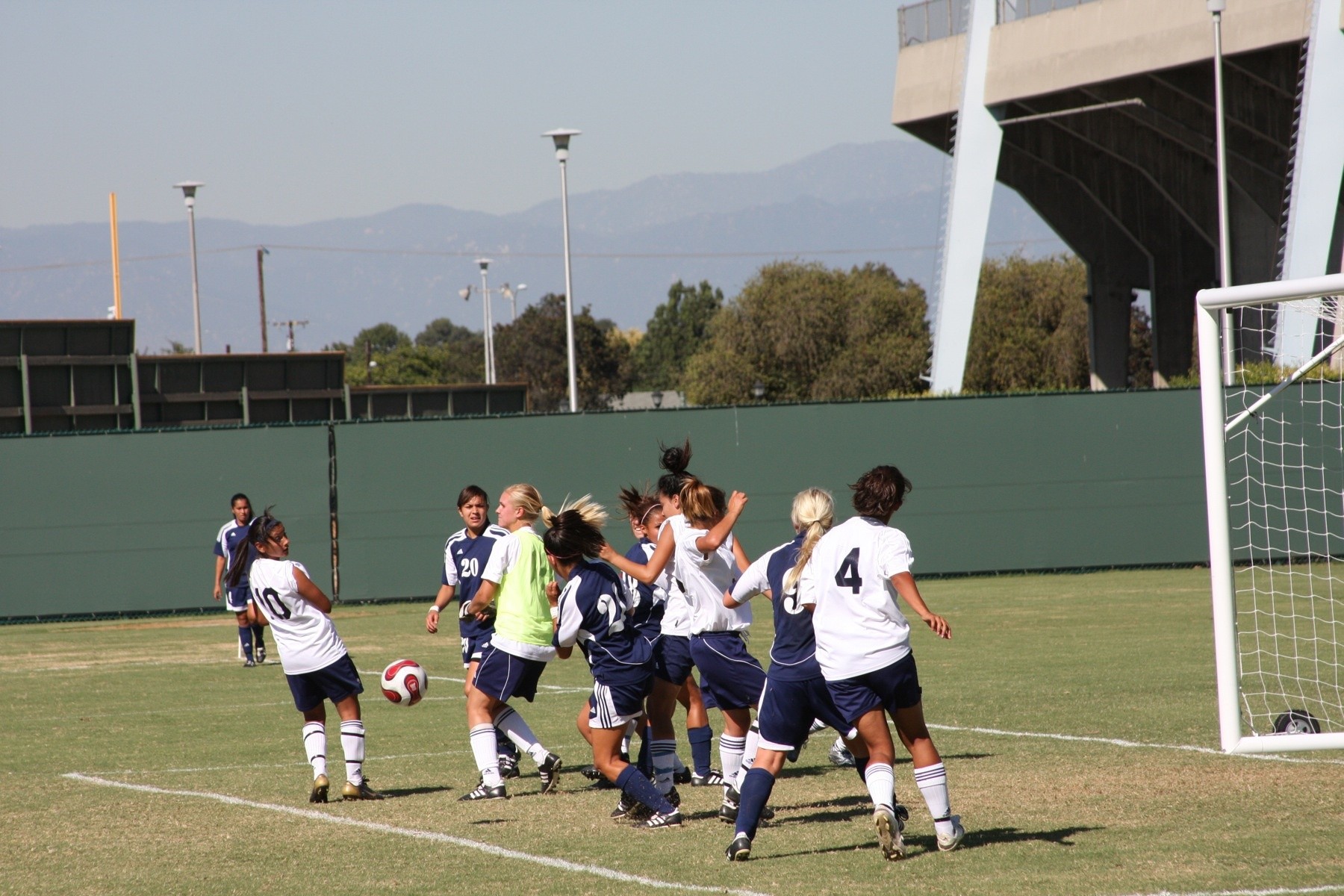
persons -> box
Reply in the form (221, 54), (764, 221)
(213, 493), (268, 667)
(425, 434), (964, 861)
(224, 503), (385, 802)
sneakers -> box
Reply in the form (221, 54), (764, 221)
(717, 802), (768, 827)
(580, 753), (630, 778)
(873, 805), (907, 860)
(725, 787), (774, 821)
(673, 767), (692, 784)
(689, 769), (724, 786)
(632, 786), (681, 819)
(635, 808), (681, 829)
(610, 776), (657, 818)
(310, 774), (329, 802)
(828, 745), (856, 767)
(725, 831), (751, 861)
(538, 753), (562, 794)
(936, 814), (965, 851)
(497, 744), (521, 777)
(255, 639), (266, 662)
(458, 781), (507, 801)
(244, 660), (256, 666)
(341, 775), (385, 802)
(588, 778), (617, 789)
(480, 767), (521, 783)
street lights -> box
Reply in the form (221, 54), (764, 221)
(540, 129), (586, 414)
(169, 182), (206, 355)
(456, 258), (528, 384)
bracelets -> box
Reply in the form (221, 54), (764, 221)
(428, 605), (439, 612)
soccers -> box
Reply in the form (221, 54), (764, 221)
(379, 659), (430, 707)
